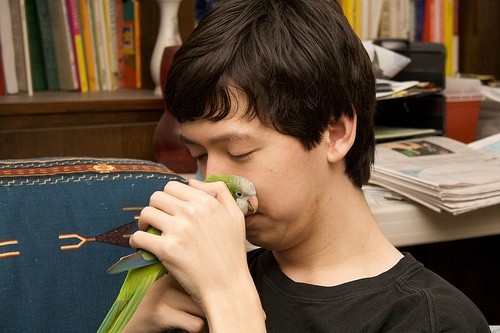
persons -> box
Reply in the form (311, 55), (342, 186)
(107, 0), (492, 333)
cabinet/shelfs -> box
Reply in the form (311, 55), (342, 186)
(0, 91), (197, 176)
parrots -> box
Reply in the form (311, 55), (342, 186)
(96, 172), (259, 333)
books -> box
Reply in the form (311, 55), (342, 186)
(0, 0), (460, 96)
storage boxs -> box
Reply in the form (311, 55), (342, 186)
(446, 77), (486, 145)
(373, 38), (447, 132)
